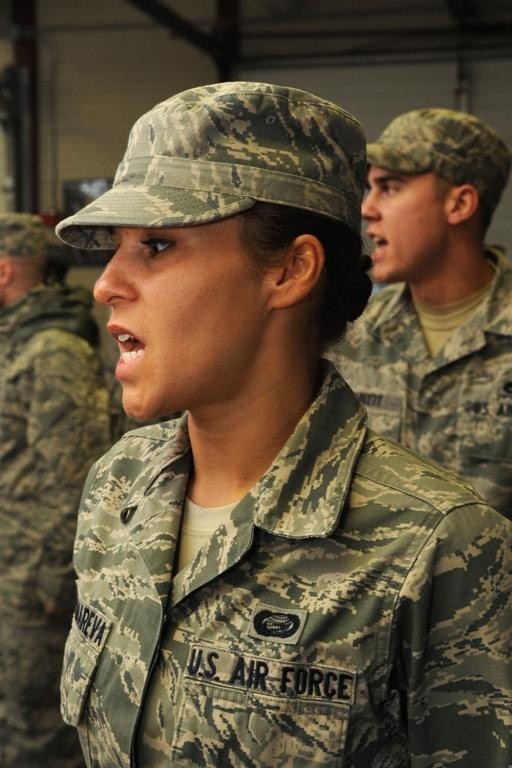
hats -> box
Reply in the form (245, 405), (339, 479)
(366, 106), (512, 213)
(54, 79), (369, 252)
(0, 211), (52, 258)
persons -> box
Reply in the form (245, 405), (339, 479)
(0, 211), (121, 766)
(53, 80), (510, 766)
(320, 109), (509, 520)
